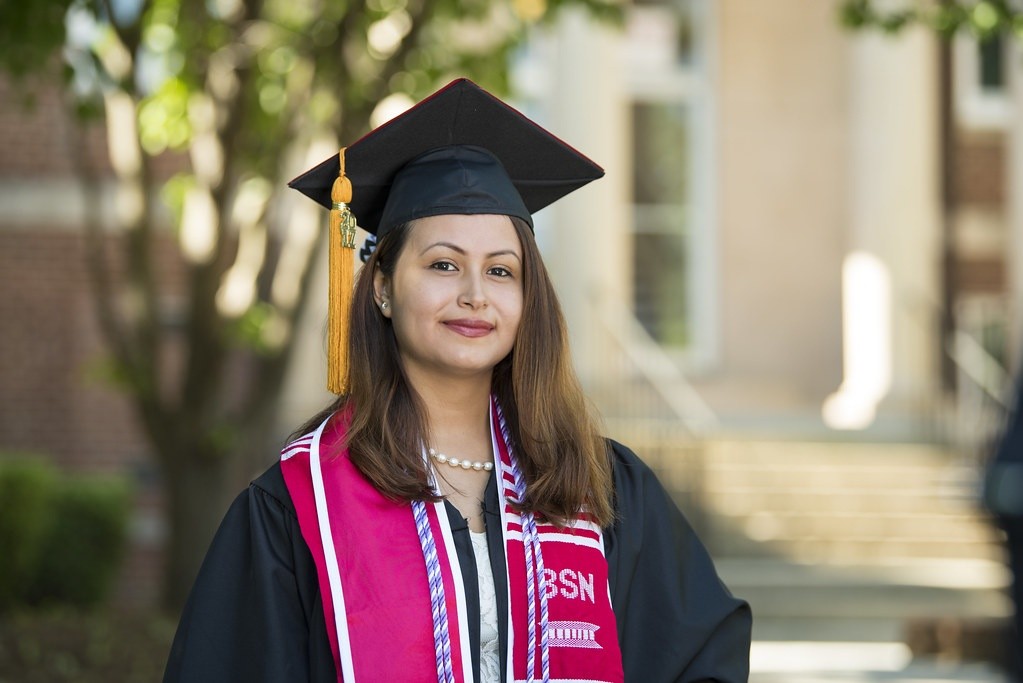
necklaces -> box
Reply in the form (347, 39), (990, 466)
(427, 447), (493, 472)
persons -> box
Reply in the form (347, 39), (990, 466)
(164, 78), (753, 683)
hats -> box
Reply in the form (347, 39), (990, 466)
(289, 76), (606, 397)
